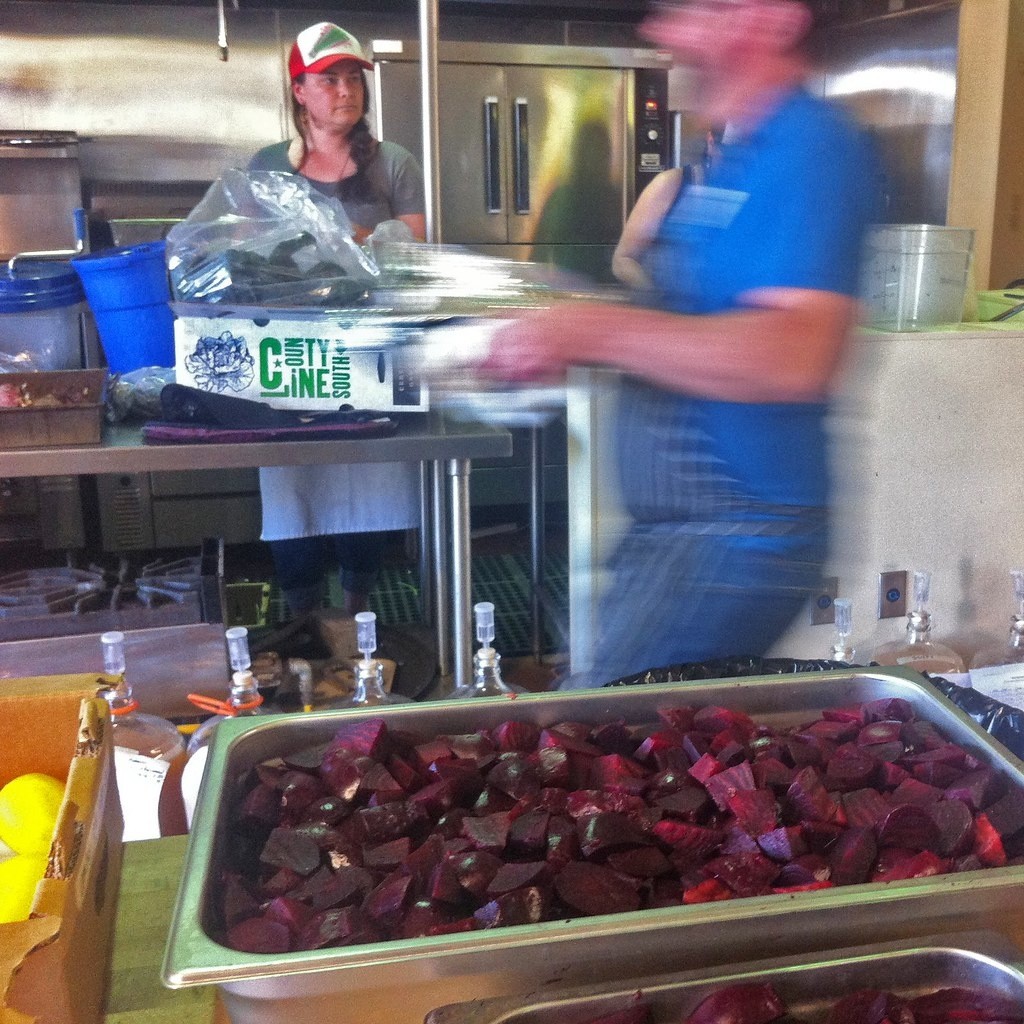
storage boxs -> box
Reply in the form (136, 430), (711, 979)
(225, 583), (271, 627)
(168, 292), (441, 418)
(0, 670), (128, 1024)
(0, 367), (109, 448)
(158, 663), (1024, 1024)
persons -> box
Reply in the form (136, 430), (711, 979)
(479, 1), (881, 690)
(235, 21), (427, 661)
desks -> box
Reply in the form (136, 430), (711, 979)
(0, 408), (518, 700)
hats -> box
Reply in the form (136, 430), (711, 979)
(290, 20), (374, 81)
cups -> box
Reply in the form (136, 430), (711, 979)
(857, 221), (978, 333)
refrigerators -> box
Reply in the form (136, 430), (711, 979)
(362, 39), (674, 291)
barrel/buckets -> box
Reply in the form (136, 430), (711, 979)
(70, 239), (176, 376)
(0, 260), (101, 374)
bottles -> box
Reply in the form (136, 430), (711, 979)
(101, 630), (188, 838)
(828, 597), (856, 666)
(877, 571), (966, 674)
(443, 601), (531, 700)
(325, 612), (415, 712)
(188, 625), (286, 762)
(968, 569), (1024, 670)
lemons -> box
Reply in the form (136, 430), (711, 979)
(0, 773), (68, 923)
